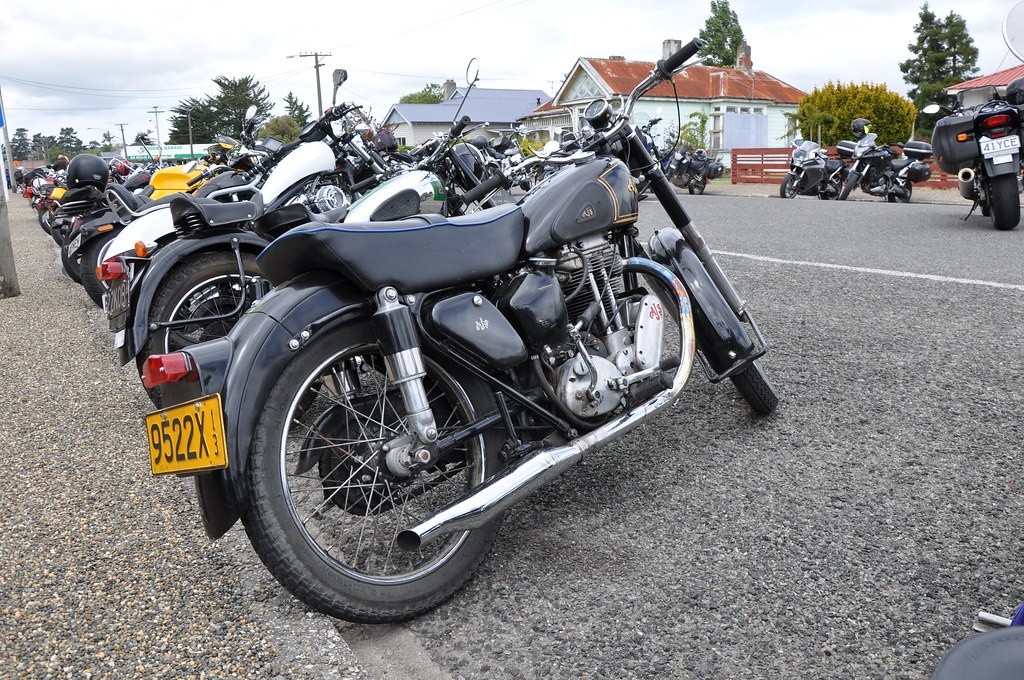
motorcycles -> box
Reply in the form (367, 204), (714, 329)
(837, 118), (934, 203)
(919, 76), (1024, 230)
(4, 34), (780, 624)
(780, 139), (857, 201)
